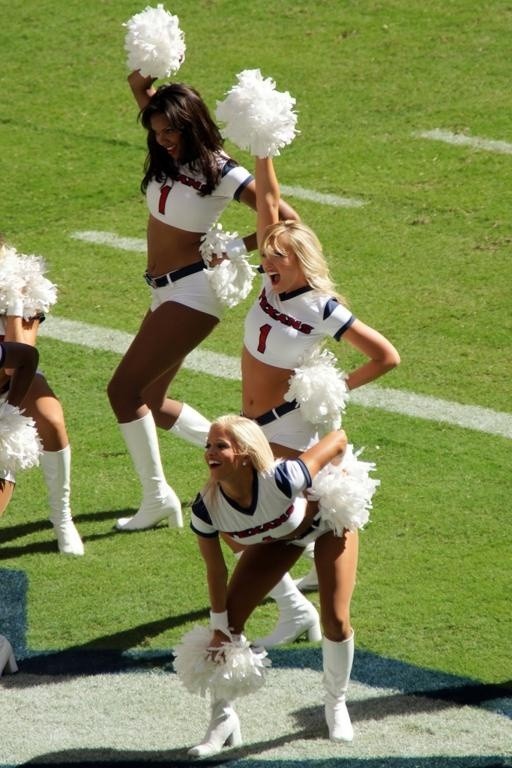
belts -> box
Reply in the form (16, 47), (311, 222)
(290, 518), (320, 540)
(144, 259), (207, 288)
(240, 399), (300, 425)
(0, 381), (10, 395)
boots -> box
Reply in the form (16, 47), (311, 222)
(167, 402), (212, 452)
(188, 556), (358, 757)
(40, 444), (83, 555)
(116, 409), (183, 530)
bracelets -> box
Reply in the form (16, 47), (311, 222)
(208, 611), (229, 631)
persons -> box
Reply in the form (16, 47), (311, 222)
(107, 62), (305, 532)
(221, 155), (402, 649)
(185, 414), (382, 756)
(0, 337), (41, 681)
(0, 243), (85, 556)
